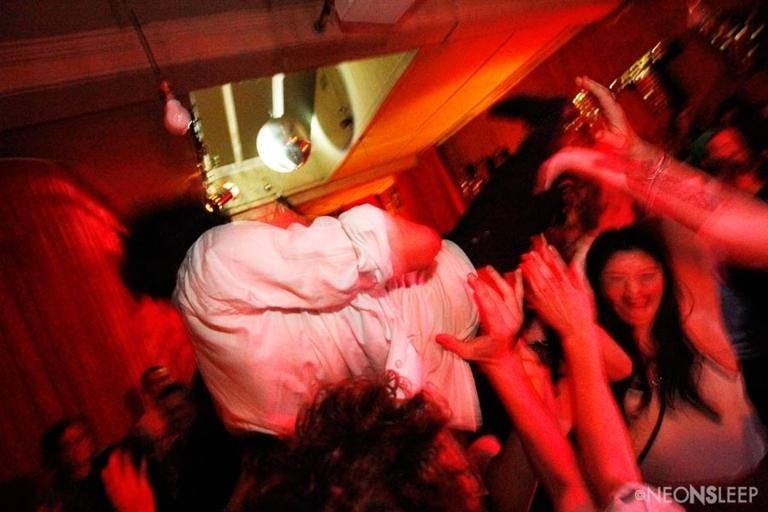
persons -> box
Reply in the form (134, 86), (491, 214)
(0, 74), (767, 512)
